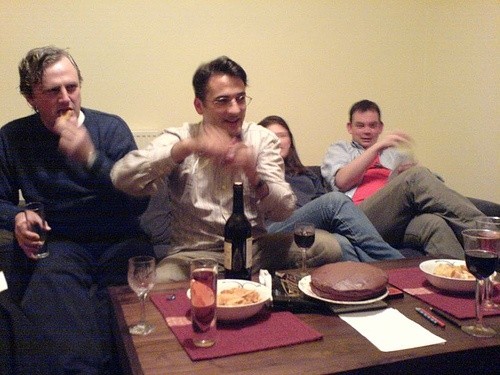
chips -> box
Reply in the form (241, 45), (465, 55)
(217, 288), (264, 306)
(433, 265), (476, 280)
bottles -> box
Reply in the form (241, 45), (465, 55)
(224, 181), (253, 282)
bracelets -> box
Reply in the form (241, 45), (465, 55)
(254, 179), (265, 190)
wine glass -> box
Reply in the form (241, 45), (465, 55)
(127, 256), (157, 335)
(293, 222), (317, 278)
(460, 216), (500, 338)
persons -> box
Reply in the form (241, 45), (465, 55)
(111, 57), (295, 284)
(0, 46), (152, 375)
(321, 99), (498, 258)
(257, 114), (405, 262)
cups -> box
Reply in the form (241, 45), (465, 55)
(24, 202), (50, 258)
(190, 258), (218, 348)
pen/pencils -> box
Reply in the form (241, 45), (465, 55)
(415, 308), (446, 328)
(430, 307), (461, 327)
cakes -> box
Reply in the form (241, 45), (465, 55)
(311, 261), (387, 301)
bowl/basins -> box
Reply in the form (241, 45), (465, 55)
(419, 258), (497, 293)
(186, 278), (271, 324)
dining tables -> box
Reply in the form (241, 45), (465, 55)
(107, 256), (500, 375)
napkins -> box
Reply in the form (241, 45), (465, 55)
(338, 308), (445, 353)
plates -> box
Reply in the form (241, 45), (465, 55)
(298, 275), (390, 305)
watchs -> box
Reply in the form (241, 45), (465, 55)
(87, 150), (96, 168)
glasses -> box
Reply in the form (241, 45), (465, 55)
(204, 95), (252, 109)
